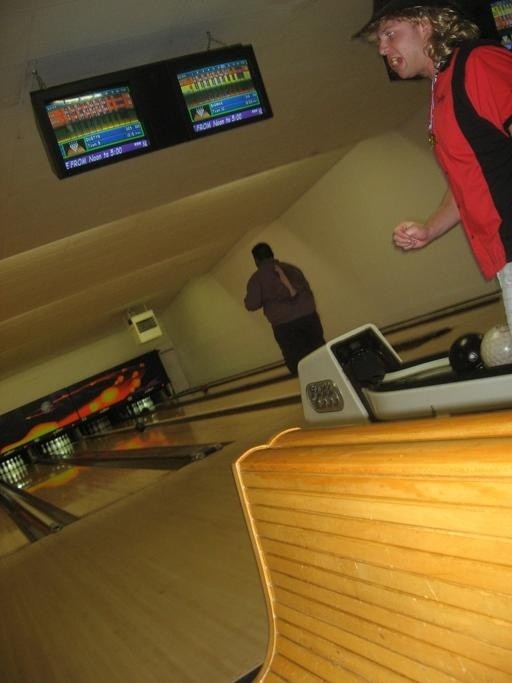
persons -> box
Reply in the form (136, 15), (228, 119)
(245, 242), (326, 378)
(349, 1), (512, 337)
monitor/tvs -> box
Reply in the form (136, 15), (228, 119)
(29, 42), (274, 180)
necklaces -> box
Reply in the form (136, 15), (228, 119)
(427, 71), (437, 147)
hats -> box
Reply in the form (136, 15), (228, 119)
(352, 2), (412, 45)
